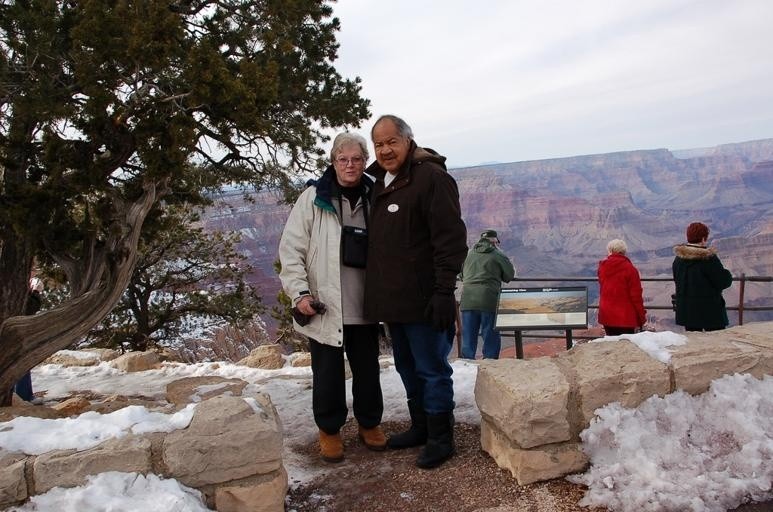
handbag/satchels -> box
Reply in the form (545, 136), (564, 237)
(341, 225), (369, 268)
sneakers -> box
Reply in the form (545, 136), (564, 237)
(359, 425), (386, 451)
(319, 429), (344, 463)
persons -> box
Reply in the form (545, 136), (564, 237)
(597, 238), (647, 337)
(365, 114), (469, 470)
(457, 230), (515, 361)
(14, 278), (45, 405)
(278, 131), (388, 463)
(671, 222), (732, 331)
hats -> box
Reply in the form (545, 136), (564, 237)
(481, 230), (501, 243)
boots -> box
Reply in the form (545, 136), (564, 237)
(387, 410), (458, 468)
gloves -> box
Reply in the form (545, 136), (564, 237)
(289, 301), (326, 327)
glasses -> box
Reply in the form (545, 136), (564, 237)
(334, 156), (364, 164)
